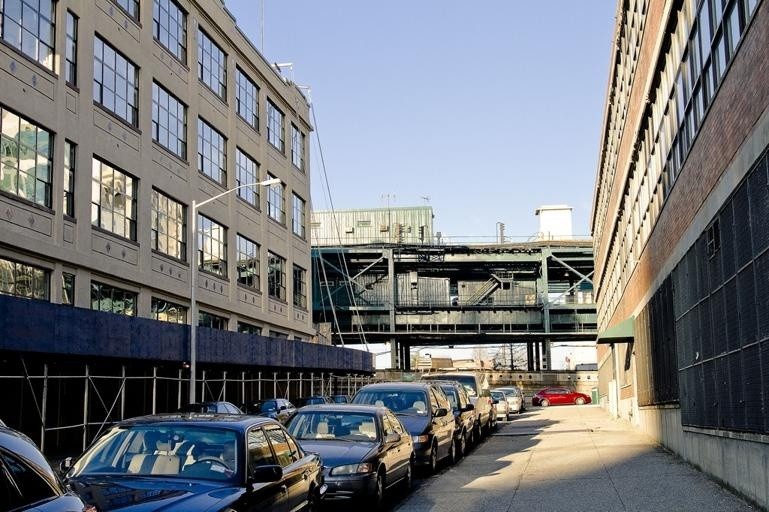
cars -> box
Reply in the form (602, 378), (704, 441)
(532, 385), (592, 406)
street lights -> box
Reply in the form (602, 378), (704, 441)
(189, 178), (282, 405)
